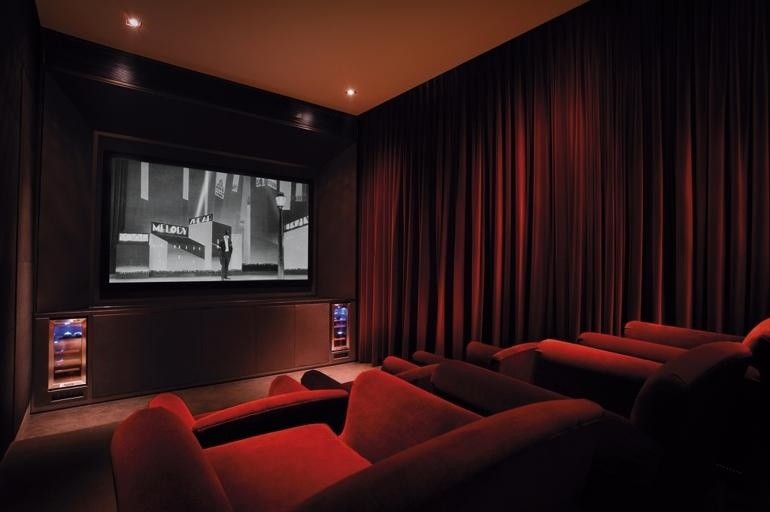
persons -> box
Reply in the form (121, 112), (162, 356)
(217, 231), (232, 279)
